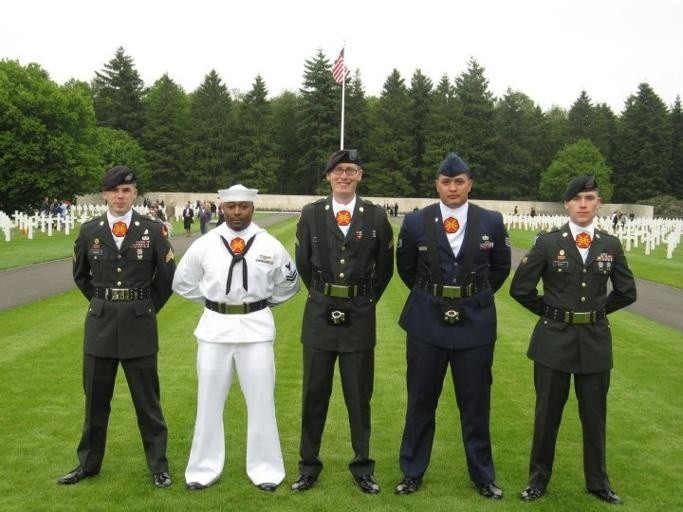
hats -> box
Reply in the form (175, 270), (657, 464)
(217, 183), (259, 204)
(563, 175), (599, 202)
(100, 165), (137, 191)
(436, 151), (471, 179)
(324, 148), (363, 174)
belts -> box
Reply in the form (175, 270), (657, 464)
(541, 303), (606, 326)
(310, 276), (372, 299)
(414, 274), (491, 300)
(203, 297), (269, 316)
(92, 285), (153, 302)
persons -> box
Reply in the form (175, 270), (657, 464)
(290, 147), (395, 495)
(509, 173), (639, 505)
(57, 163), (177, 488)
(612, 209), (634, 232)
(384, 203), (398, 217)
(515, 206), (517, 214)
(171, 182), (302, 494)
(393, 150), (511, 500)
(143, 198), (167, 221)
(182, 197), (225, 235)
(531, 208), (535, 217)
(41, 197), (68, 217)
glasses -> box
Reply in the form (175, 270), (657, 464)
(329, 166), (359, 176)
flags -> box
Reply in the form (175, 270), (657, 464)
(331, 47), (352, 85)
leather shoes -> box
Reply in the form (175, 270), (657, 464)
(474, 481), (504, 500)
(56, 465), (97, 486)
(256, 481), (279, 494)
(584, 486), (624, 505)
(186, 481), (205, 491)
(354, 473), (380, 494)
(395, 476), (424, 496)
(289, 473), (318, 491)
(151, 470), (172, 489)
(519, 485), (547, 502)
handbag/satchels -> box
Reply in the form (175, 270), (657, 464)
(190, 218), (195, 224)
